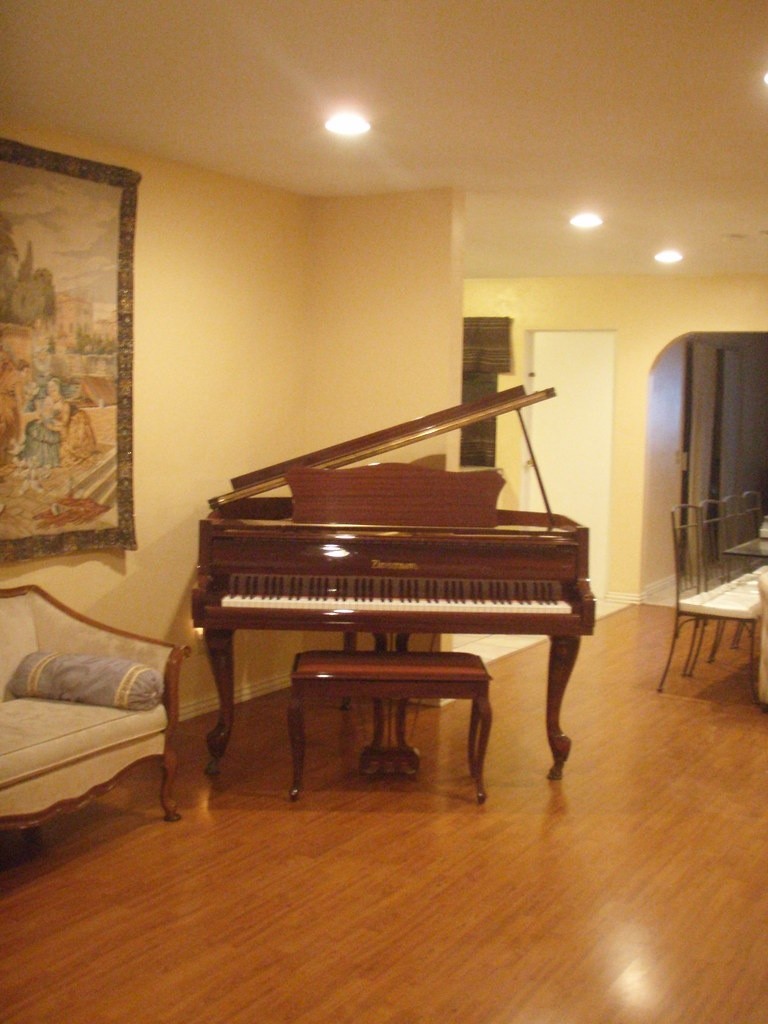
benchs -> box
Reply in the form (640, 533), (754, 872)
(290, 651), (492, 805)
(657, 489), (762, 705)
(0, 584), (191, 832)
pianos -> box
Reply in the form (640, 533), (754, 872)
(190, 383), (596, 805)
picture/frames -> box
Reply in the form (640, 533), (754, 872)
(0, 135), (142, 566)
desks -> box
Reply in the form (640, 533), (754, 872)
(721, 537), (768, 708)
